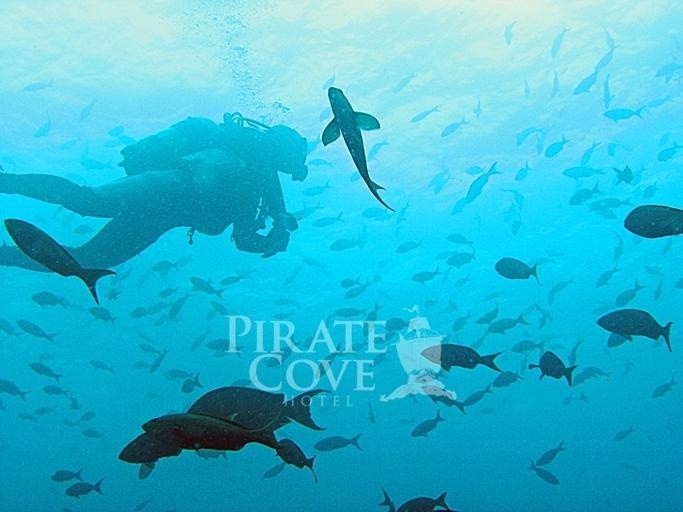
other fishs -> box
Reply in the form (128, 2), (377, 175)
(0, 19), (682, 512)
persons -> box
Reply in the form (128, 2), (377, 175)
(0, 123), (308, 275)
